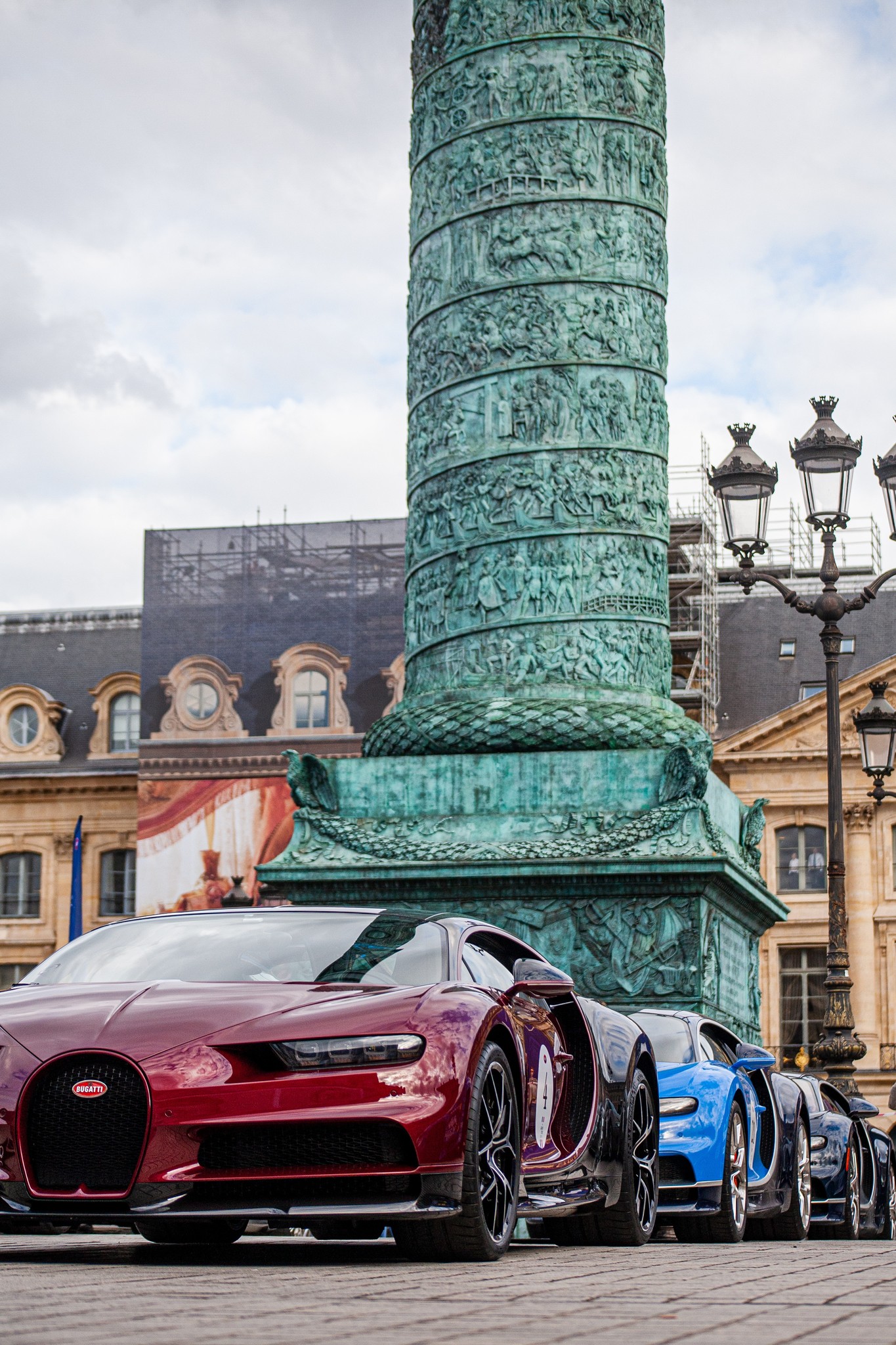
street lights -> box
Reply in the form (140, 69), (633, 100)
(699, 394), (895, 1094)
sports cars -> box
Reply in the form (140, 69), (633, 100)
(0, 902), (666, 1262)
(593, 1006), (817, 1242)
(777, 1070), (896, 1242)
